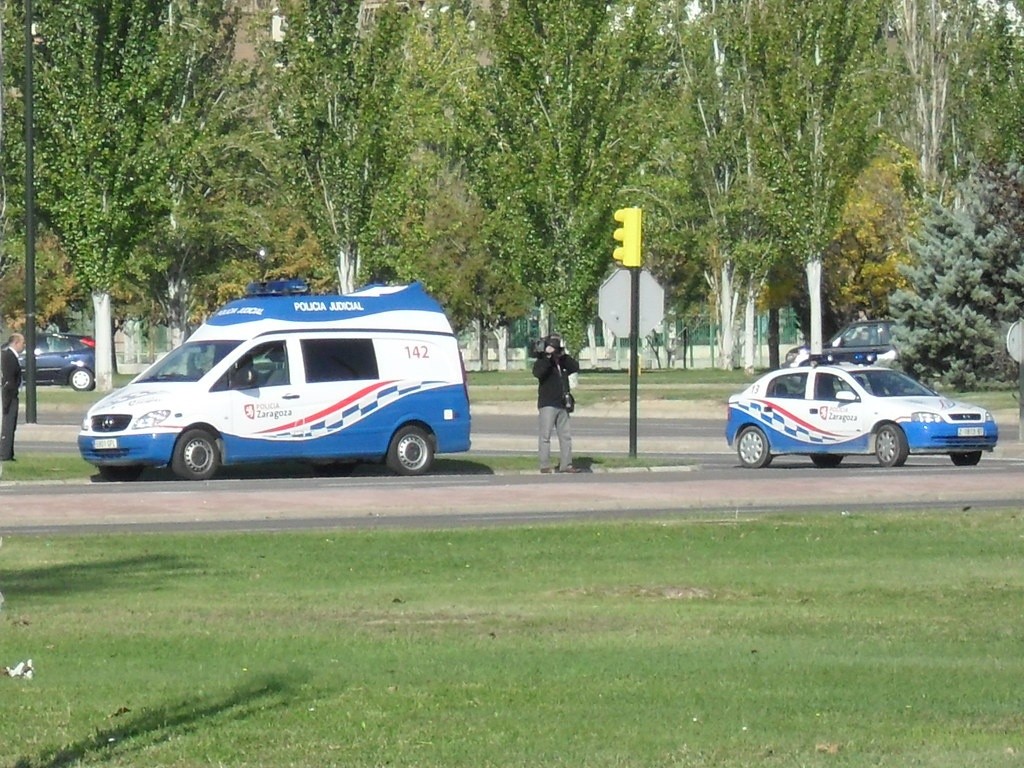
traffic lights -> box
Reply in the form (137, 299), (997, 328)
(613, 207), (643, 268)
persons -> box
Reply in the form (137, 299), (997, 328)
(532, 334), (581, 475)
(0, 333), (26, 461)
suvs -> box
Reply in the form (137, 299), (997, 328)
(777, 320), (898, 371)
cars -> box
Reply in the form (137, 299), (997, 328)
(0, 331), (94, 392)
(725, 364), (998, 468)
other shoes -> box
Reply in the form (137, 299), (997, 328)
(558, 463), (581, 471)
(541, 466), (553, 474)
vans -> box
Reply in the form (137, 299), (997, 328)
(76, 280), (472, 476)
